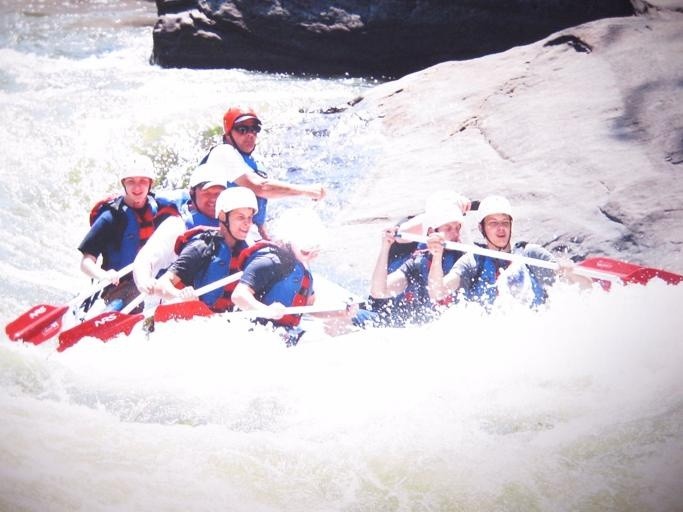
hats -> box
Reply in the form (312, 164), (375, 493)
(235, 114), (263, 127)
(202, 177), (226, 189)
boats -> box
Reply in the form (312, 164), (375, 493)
(294, 297), (458, 338)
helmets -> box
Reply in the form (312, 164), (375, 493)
(120, 155), (157, 181)
(422, 202), (463, 237)
(223, 106), (262, 133)
(214, 186), (257, 220)
(190, 164), (226, 190)
(476, 196), (513, 226)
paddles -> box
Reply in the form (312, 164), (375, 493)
(155, 301), (351, 321)
(394, 231), (681, 286)
(573, 256), (644, 289)
(58, 271), (242, 350)
(5, 263), (132, 344)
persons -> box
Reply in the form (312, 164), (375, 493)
(189, 104), (326, 239)
(352, 198), (473, 329)
(132, 163), (226, 321)
(152, 187), (259, 315)
(77, 155), (176, 313)
(231, 227), (322, 347)
(425, 195), (575, 313)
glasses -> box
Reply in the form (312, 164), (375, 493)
(233, 127), (262, 135)
